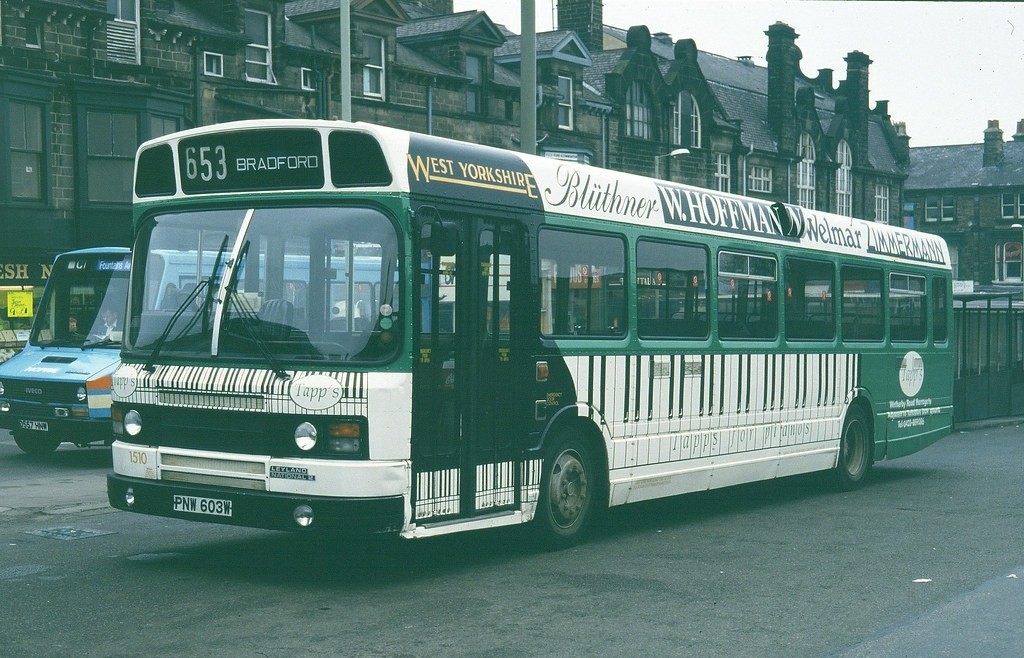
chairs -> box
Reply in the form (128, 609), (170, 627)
(256, 298), (295, 339)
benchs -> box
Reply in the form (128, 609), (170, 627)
(637, 310), (924, 338)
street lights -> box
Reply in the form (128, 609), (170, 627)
(652, 147), (691, 180)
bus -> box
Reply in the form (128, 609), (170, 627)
(0, 244), (438, 458)
(104, 116), (956, 555)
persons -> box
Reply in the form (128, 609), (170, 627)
(92, 307), (124, 339)
